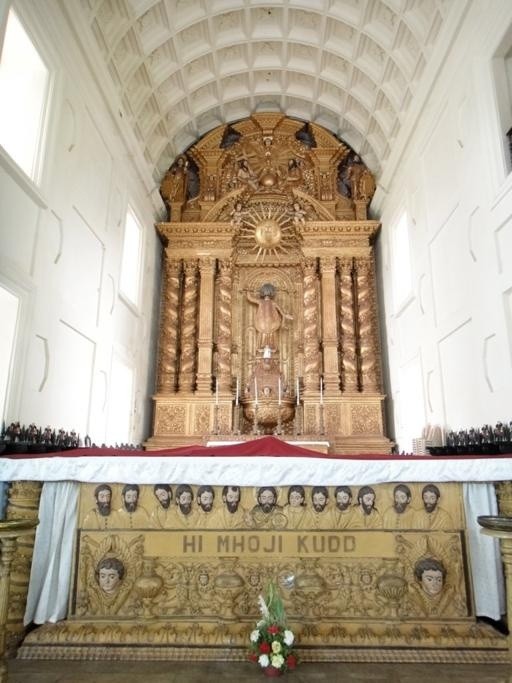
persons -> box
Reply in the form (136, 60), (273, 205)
(414, 558), (448, 598)
(245, 283), (294, 355)
(167, 137), (363, 229)
(95, 559), (123, 593)
(195, 567), (377, 595)
(81, 482), (457, 529)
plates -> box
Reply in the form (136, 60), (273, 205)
(477, 515), (512, 532)
(0, 518), (40, 531)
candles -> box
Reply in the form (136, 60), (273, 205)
(255, 378), (257, 401)
(216, 379), (218, 404)
(279, 378), (281, 405)
(297, 378), (299, 405)
(236, 379), (238, 405)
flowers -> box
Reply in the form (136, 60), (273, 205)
(249, 581), (300, 673)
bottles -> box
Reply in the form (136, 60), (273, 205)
(295, 555), (327, 623)
(134, 555), (165, 619)
(212, 554), (247, 623)
(374, 556), (409, 620)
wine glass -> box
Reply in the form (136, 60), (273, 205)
(239, 399), (295, 435)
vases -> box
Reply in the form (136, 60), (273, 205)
(262, 667), (280, 676)
